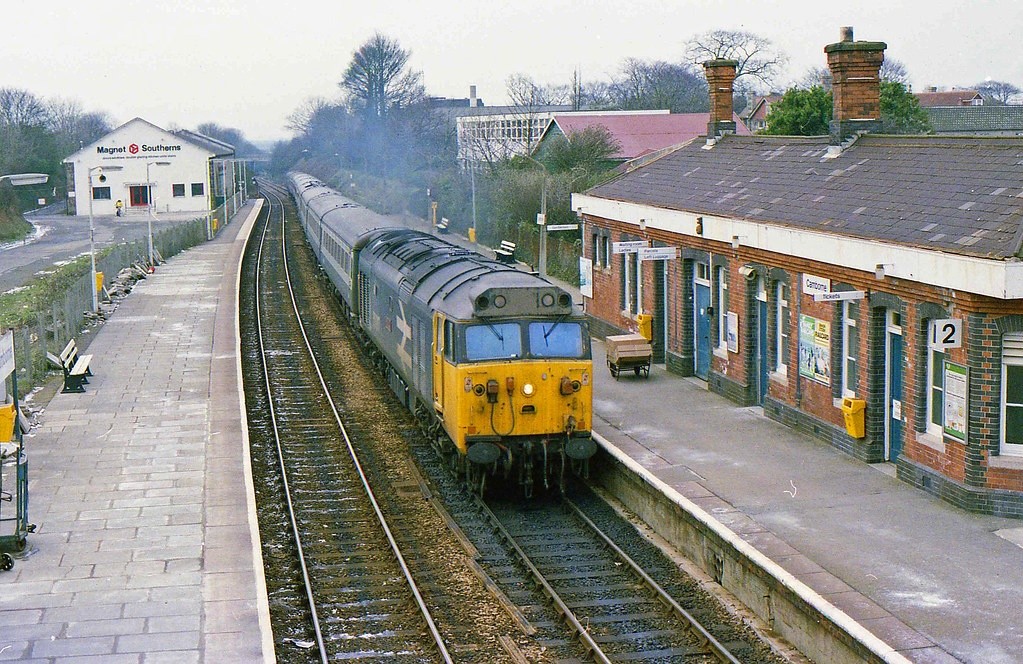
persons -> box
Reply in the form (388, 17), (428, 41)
(116, 200), (123, 217)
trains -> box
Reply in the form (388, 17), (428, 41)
(284, 169), (597, 498)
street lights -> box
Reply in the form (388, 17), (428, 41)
(88, 166), (123, 313)
(457, 157), (477, 230)
(516, 148), (548, 276)
(147, 161), (171, 266)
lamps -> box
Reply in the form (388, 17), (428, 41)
(876, 261), (896, 283)
(730, 234), (747, 249)
(576, 205), (588, 217)
(638, 217), (652, 230)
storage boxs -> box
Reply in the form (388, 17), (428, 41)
(605, 334), (653, 363)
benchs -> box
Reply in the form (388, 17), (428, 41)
(60, 339), (94, 394)
(437, 218), (449, 232)
(493, 240), (516, 263)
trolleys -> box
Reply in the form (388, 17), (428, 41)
(605, 332), (652, 382)
